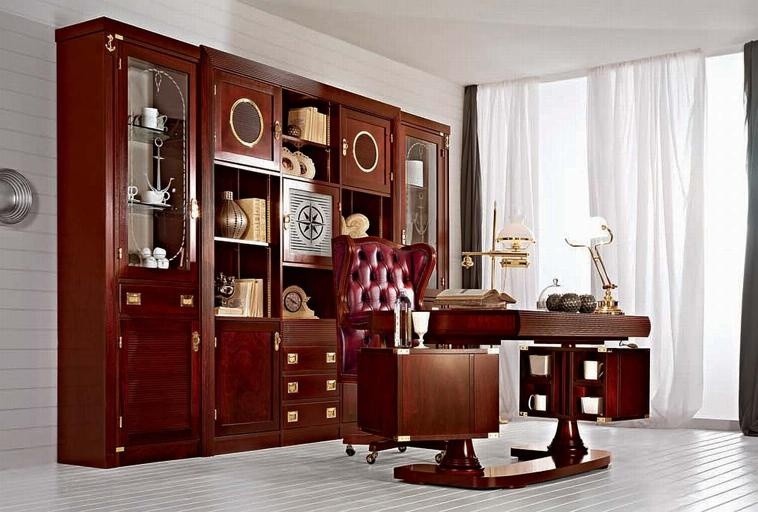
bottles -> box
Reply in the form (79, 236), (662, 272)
(394, 287), (413, 348)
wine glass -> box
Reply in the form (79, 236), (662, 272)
(411, 311), (431, 348)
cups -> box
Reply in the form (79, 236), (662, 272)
(141, 190), (170, 203)
(141, 107), (159, 129)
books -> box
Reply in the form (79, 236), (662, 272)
(285, 107), (328, 147)
(435, 287), (518, 311)
(234, 197), (268, 243)
(214, 277), (266, 319)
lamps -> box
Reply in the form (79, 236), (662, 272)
(564, 216), (625, 314)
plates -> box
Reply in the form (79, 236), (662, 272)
(143, 202), (171, 208)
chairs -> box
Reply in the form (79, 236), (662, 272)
(331, 234), (450, 465)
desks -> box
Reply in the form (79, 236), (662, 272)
(393, 310), (651, 490)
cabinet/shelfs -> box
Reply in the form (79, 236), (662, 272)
(357, 348), (499, 442)
(54, 16), (449, 469)
